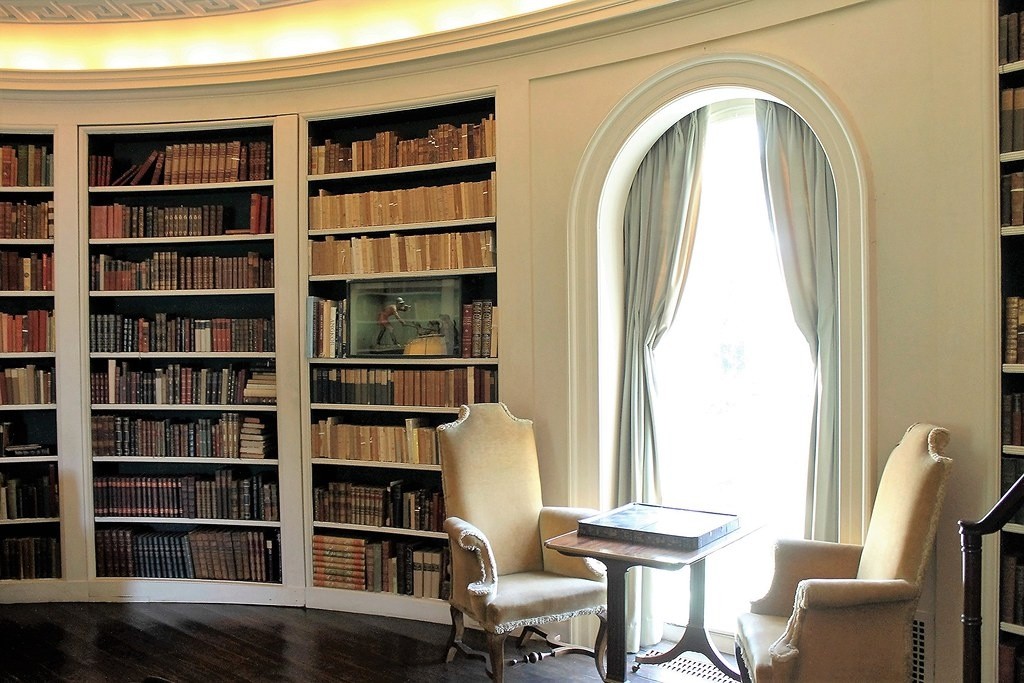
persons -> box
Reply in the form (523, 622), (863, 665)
(376, 298), (410, 348)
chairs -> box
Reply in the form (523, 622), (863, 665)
(436, 403), (608, 683)
(733, 423), (953, 683)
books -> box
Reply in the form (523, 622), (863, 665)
(998, 11), (1024, 683)
(0, 113), (498, 599)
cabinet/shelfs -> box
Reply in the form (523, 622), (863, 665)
(0, 84), (499, 628)
(989, 0), (1024, 683)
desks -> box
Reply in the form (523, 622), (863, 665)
(543, 522), (761, 683)
